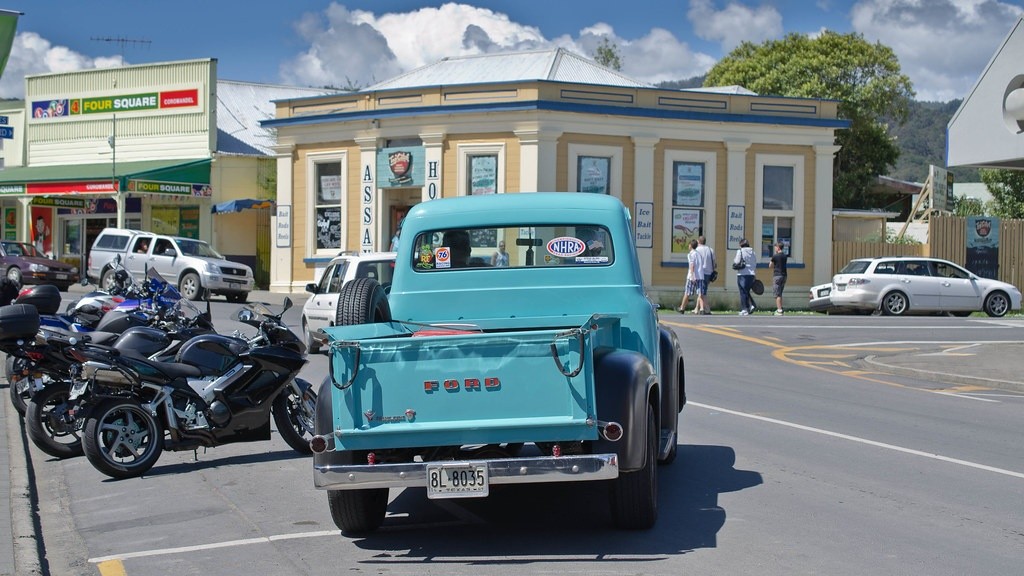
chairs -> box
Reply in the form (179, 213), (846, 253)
(568, 229), (606, 263)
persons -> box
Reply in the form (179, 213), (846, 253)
(491, 240), (509, 267)
(675, 240), (706, 315)
(137, 239), (149, 254)
(734, 238), (758, 315)
(438, 231), (472, 268)
(769, 242), (787, 316)
(691, 236), (717, 315)
(390, 228), (399, 252)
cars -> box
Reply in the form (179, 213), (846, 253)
(0, 239), (78, 292)
(829, 255), (1023, 318)
(809, 264), (914, 316)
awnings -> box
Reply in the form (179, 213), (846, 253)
(0, 156), (211, 241)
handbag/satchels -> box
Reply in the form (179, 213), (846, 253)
(710, 271), (717, 282)
(732, 249), (746, 270)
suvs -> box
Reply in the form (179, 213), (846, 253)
(300, 249), (419, 354)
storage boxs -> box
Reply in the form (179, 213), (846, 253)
(14, 286), (62, 313)
(0, 304), (40, 341)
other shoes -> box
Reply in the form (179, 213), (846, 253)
(691, 308), (698, 314)
(705, 311), (711, 315)
(700, 309), (705, 315)
(749, 304), (755, 313)
(674, 306), (684, 314)
(774, 308), (783, 316)
(739, 309), (748, 316)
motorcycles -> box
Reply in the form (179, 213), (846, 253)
(0, 286), (221, 459)
(0, 253), (202, 422)
(46, 296), (318, 478)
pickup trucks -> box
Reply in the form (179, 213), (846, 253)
(308, 191), (688, 535)
(87, 227), (255, 303)
